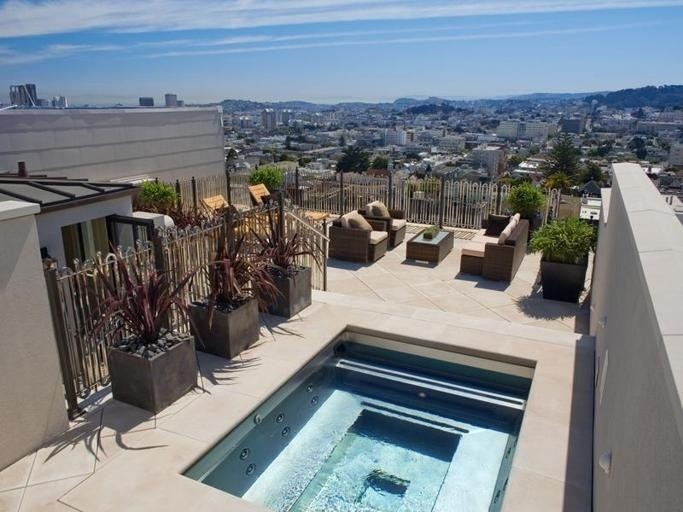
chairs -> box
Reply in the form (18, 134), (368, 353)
(329, 200), (405, 266)
(197, 183), (328, 255)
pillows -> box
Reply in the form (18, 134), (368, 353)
(484, 213), (511, 237)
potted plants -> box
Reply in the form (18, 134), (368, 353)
(528, 215), (596, 305)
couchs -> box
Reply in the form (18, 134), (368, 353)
(458, 212), (529, 283)
